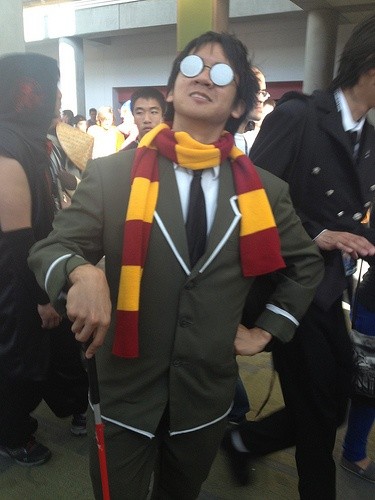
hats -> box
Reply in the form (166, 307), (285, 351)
(55, 122), (94, 171)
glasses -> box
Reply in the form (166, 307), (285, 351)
(257, 91), (269, 101)
(175, 55), (239, 91)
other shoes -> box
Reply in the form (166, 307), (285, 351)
(339, 455), (375, 483)
(221, 429), (254, 486)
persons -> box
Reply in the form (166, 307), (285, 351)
(39, 98), (139, 433)
(0, 52), (90, 467)
(220, 9), (375, 500)
(337, 267), (375, 484)
(26, 30), (325, 499)
(119, 86), (168, 154)
(232, 66), (279, 160)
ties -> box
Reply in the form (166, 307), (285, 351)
(349, 131), (357, 154)
(184, 169), (207, 268)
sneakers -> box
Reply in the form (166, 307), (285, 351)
(70, 412), (87, 435)
(0, 437), (50, 466)
